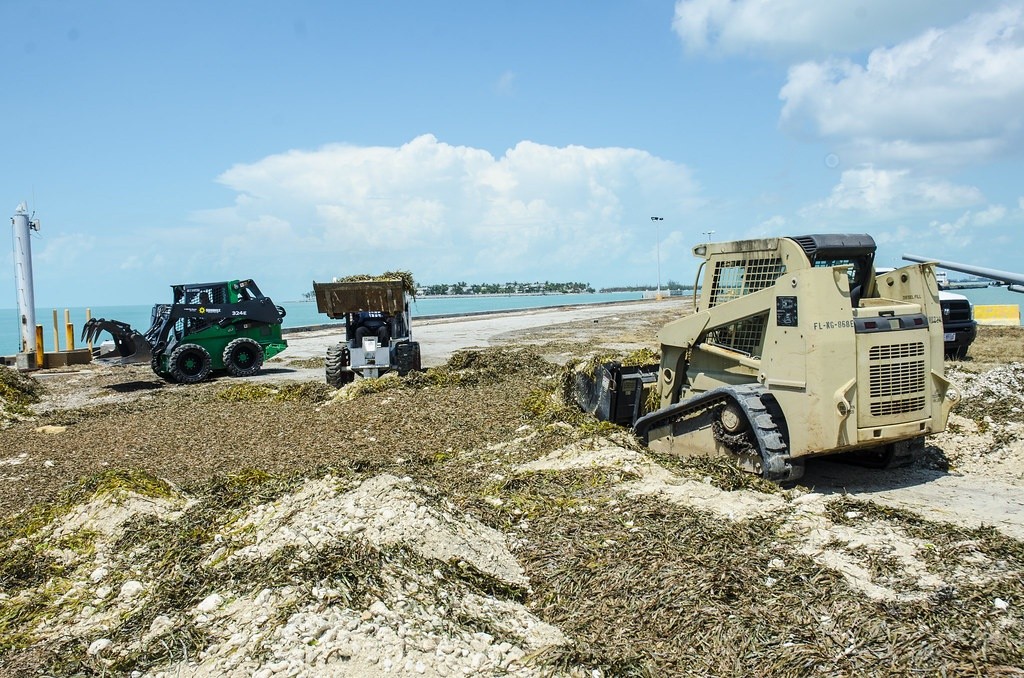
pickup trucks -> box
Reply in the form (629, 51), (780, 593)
(846, 268), (978, 360)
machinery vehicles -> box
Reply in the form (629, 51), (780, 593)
(571, 234), (961, 490)
(311, 271), (423, 389)
(80, 278), (288, 385)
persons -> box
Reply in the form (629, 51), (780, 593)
(718, 270), (774, 350)
(188, 293), (213, 331)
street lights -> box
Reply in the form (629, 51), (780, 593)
(651, 216), (664, 301)
